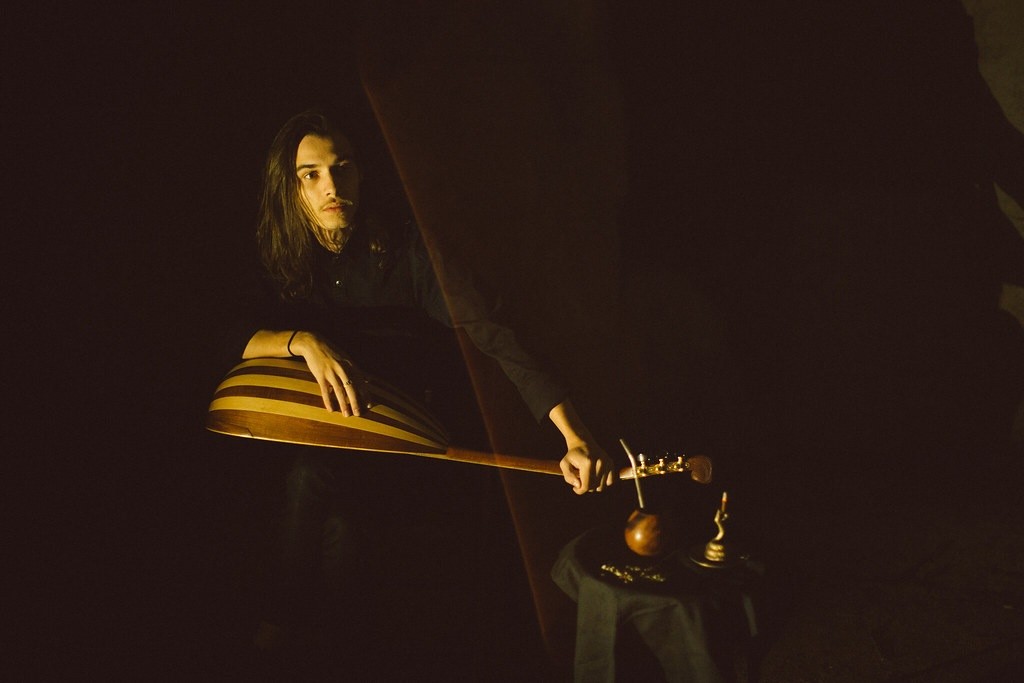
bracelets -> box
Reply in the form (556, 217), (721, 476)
(287, 328), (300, 358)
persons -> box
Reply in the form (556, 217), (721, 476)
(215, 107), (616, 683)
